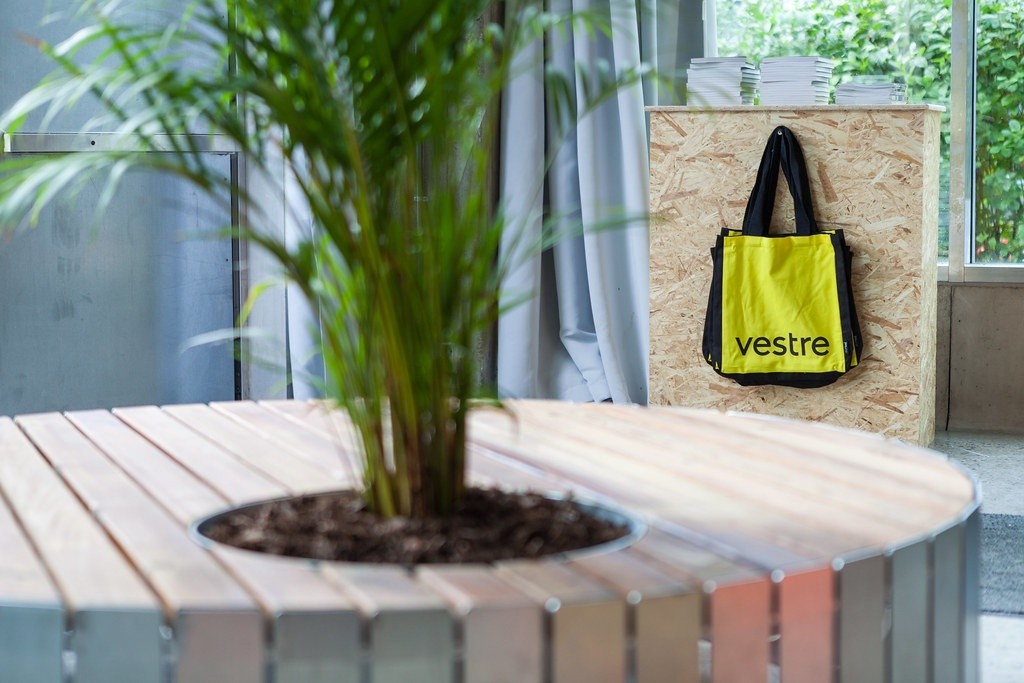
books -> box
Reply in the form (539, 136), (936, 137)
(686, 56), (908, 106)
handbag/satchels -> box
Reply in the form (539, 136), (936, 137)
(702, 125), (864, 389)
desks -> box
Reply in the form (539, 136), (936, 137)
(643, 102), (946, 449)
